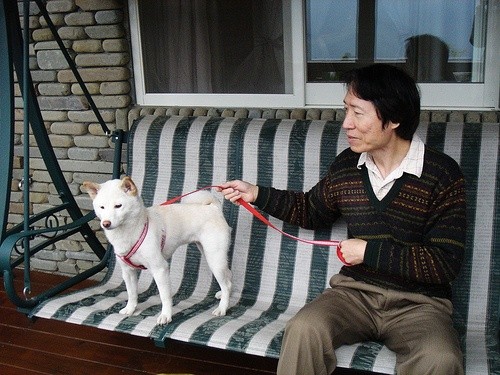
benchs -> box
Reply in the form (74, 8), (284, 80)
(0, 115), (499, 375)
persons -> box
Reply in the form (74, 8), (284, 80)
(402, 34), (449, 71)
(215, 65), (470, 375)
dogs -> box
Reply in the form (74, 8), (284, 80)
(82, 177), (234, 327)
